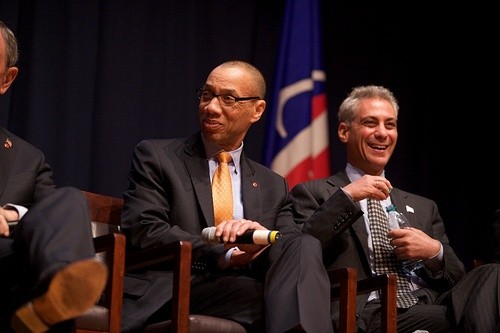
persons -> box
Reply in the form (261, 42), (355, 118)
(289, 85), (500, 333)
(0, 21), (109, 333)
(112, 60), (334, 333)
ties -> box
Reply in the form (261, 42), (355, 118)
(367, 194), (419, 309)
(211, 151), (235, 226)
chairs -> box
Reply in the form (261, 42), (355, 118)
(76, 190), (397, 333)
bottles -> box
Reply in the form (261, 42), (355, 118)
(386, 204), (424, 273)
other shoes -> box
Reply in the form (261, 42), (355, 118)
(12, 256), (108, 333)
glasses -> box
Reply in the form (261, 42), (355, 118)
(196, 88), (261, 106)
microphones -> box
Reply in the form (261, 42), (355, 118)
(201, 226), (282, 245)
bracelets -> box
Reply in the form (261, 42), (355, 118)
(421, 239), (441, 262)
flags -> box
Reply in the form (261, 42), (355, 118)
(260, 0), (330, 192)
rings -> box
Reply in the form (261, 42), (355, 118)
(376, 181), (378, 189)
